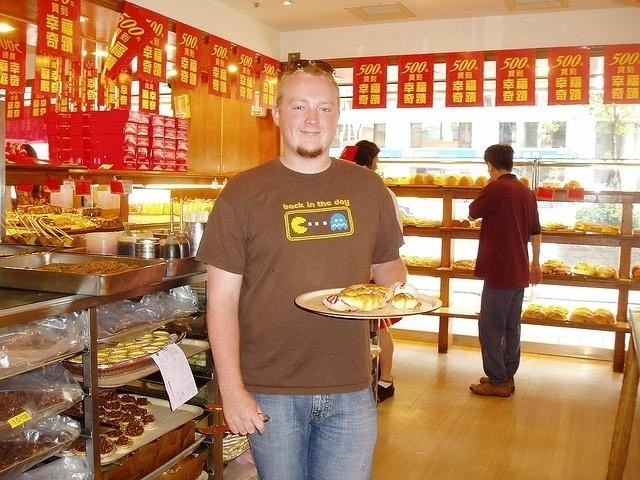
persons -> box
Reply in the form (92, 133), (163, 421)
(467, 143), (543, 398)
(193, 57), (407, 479)
(367, 185), (405, 403)
(350, 139), (381, 172)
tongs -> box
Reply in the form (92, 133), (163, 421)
(198, 403), (271, 436)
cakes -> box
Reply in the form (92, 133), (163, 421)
(63, 391), (160, 458)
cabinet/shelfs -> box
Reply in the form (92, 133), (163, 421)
(385, 187), (639, 371)
(1, 269), (226, 479)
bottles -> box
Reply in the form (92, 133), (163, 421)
(117, 232), (189, 261)
(91, 183), (100, 206)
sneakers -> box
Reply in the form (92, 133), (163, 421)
(376, 380), (397, 403)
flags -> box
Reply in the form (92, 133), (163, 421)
(352, 56), (388, 109)
(495, 48), (536, 107)
(397, 54), (435, 108)
(445, 52), (484, 107)
(602, 44), (640, 105)
(546, 46), (592, 106)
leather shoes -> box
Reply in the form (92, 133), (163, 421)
(478, 375), (516, 393)
(469, 380), (513, 398)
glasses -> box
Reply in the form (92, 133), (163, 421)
(286, 59), (337, 77)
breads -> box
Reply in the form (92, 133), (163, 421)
(522, 303), (617, 326)
(391, 292), (420, 310)
(1, 392), (64, 420)
(129, 197), (217, 218)
(2, 203), (125, 246)
(0, 430), (72, 472)
(321, 281), (395, 312)
(386, 174), (640, 282)
(0, 335), (57, 351)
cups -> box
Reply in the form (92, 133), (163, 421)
(58, 183), (74, 208)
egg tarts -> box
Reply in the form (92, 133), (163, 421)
(69, 330), (171, 365)
